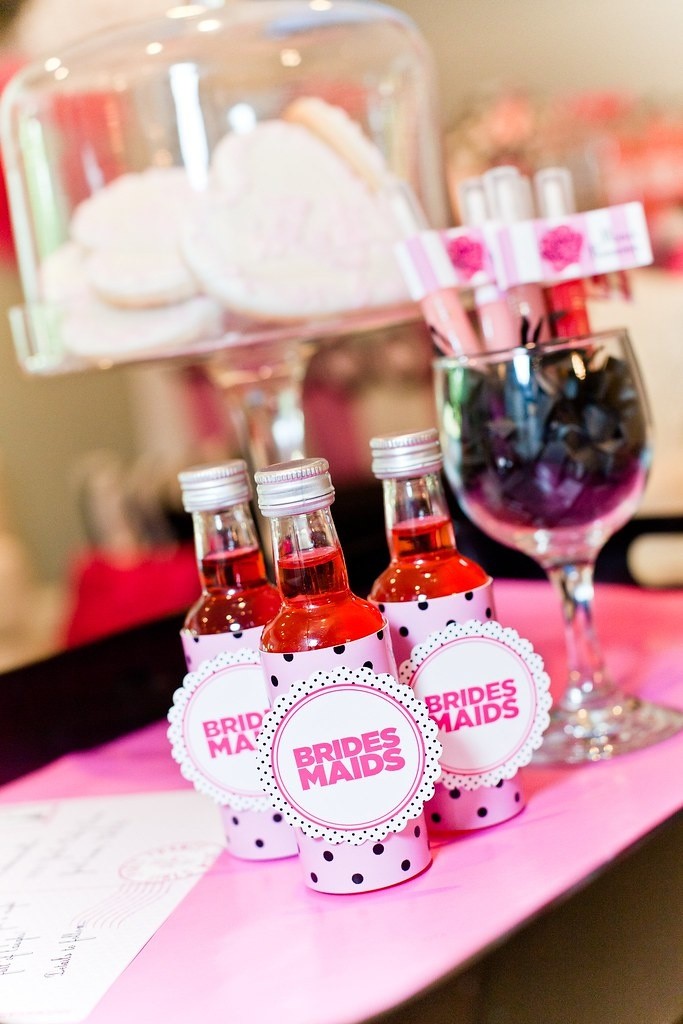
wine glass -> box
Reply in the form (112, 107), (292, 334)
(431, 327), (683, 764)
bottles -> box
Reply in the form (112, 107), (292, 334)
(253, 457), (431, 895)
(176, 460), (299, 861)
(367, 427), (527, 829)
